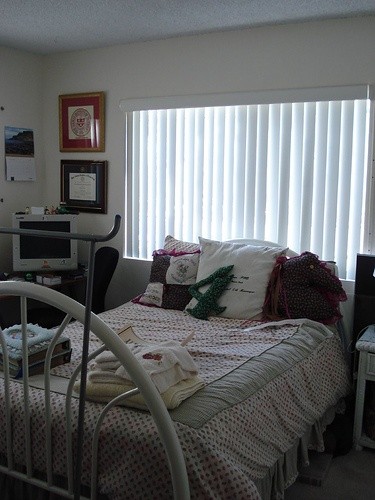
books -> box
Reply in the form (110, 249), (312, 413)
(0, 349), (72, 377)
(1, 323), (71, 369)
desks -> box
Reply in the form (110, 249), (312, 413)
(0, 263), (85, 320)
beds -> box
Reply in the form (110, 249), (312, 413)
(1, 239), (353, 499)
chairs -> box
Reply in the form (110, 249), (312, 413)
(84, 246), (119, 314)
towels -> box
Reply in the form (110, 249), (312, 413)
(95, 341), (143, 370)
(113, 339), (198, 393)
(88, 368), (134, 385)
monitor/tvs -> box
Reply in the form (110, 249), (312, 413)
(12, 213), (78, 271)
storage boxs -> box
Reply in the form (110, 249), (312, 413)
(37, 275), (62, 285)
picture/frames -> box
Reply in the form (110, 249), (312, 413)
(58, 91), (106, 153)
(59, 159), (108, 215)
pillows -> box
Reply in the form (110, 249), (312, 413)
(131, 247), (200, 310)
(263, 250), (348, 325)
(184, 236), (289, 322)
(163, 234), (201, 253)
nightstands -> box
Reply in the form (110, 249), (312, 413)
(353, 322), (375, 451)
